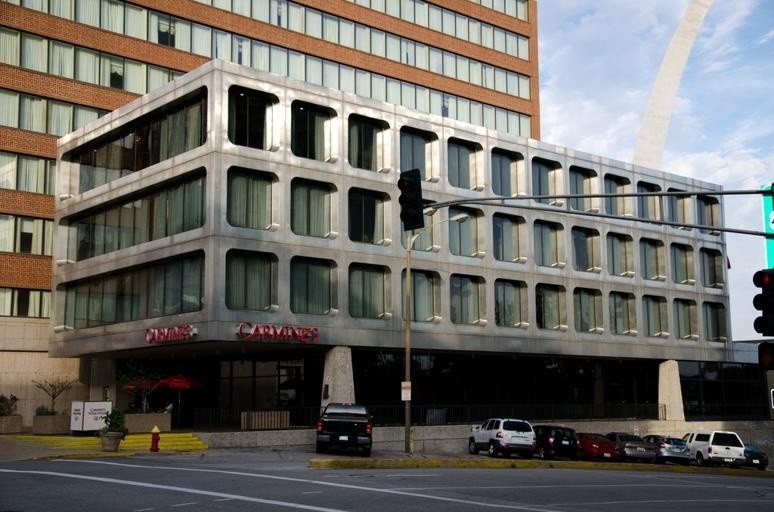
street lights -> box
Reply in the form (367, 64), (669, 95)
(401, 207), (468, 453)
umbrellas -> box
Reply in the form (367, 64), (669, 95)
(121, 378), (162, 413)
(159, 373), (202, 416)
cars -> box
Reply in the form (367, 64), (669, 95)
(605, 432), (656, 462)
(642, 434), (690, 465)
(729, 443), (768, 471)
(575, 432), (617, 460)
(533, 423), (581, 462)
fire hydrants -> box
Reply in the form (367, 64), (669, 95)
(148, 425), (160, 452)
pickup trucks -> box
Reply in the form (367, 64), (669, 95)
(314, 402), (375, 457)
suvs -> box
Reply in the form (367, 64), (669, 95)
(679, 430), (746, 470)
(467, 417), (534, 458)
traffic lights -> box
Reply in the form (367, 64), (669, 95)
(753, 268), (773, 336)
(397, 169), (426, 232)
(757, 343), (773, 370)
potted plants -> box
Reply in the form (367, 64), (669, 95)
(31, 376), (78, 434)
(0, 394), (23, 434)
(99, 409), (126, 452)
